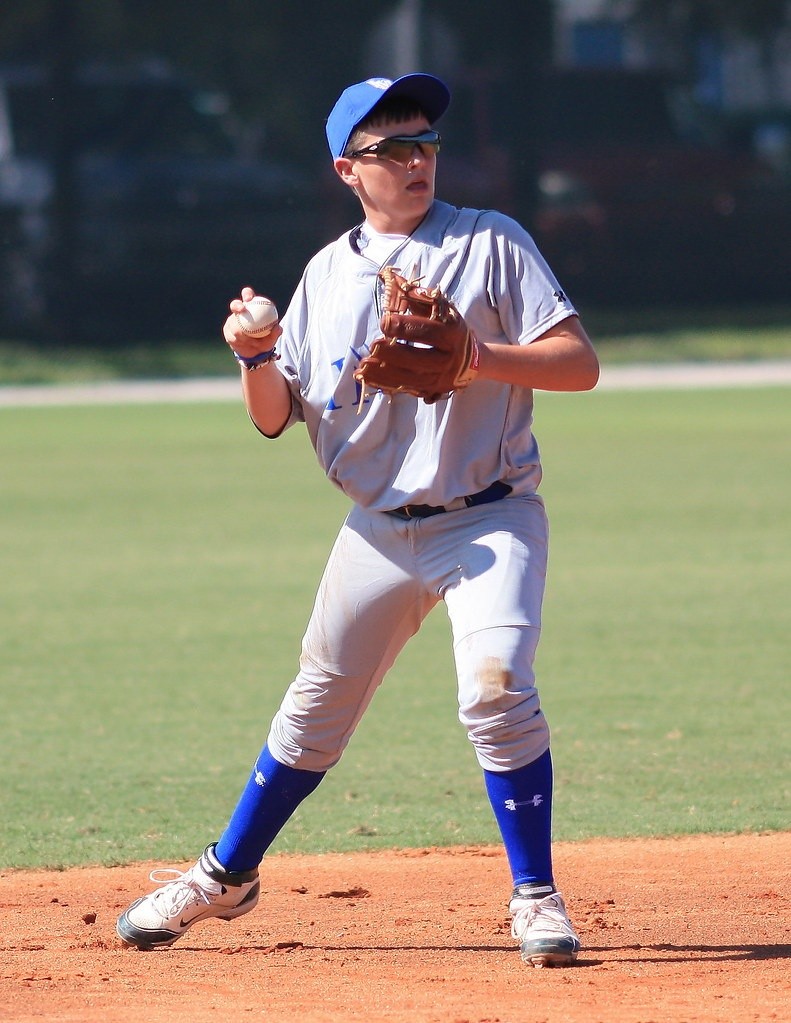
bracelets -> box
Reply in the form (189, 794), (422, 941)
(232, 348), (275, 372)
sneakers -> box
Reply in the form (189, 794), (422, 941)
(116, 841), (259, 948)
(508, 880), (579, 968)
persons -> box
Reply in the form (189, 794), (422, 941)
(116, 73), (600, 966)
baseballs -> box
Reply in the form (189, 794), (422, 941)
(236, 296), (277, 339)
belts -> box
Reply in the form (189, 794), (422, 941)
(383, 480), (513, 521)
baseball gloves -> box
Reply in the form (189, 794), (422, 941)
(354, 264), (478, 403)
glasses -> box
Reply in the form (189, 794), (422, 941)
(342, 129), (442, 162)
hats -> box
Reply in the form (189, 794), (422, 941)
(325, 73), (451, 161)
